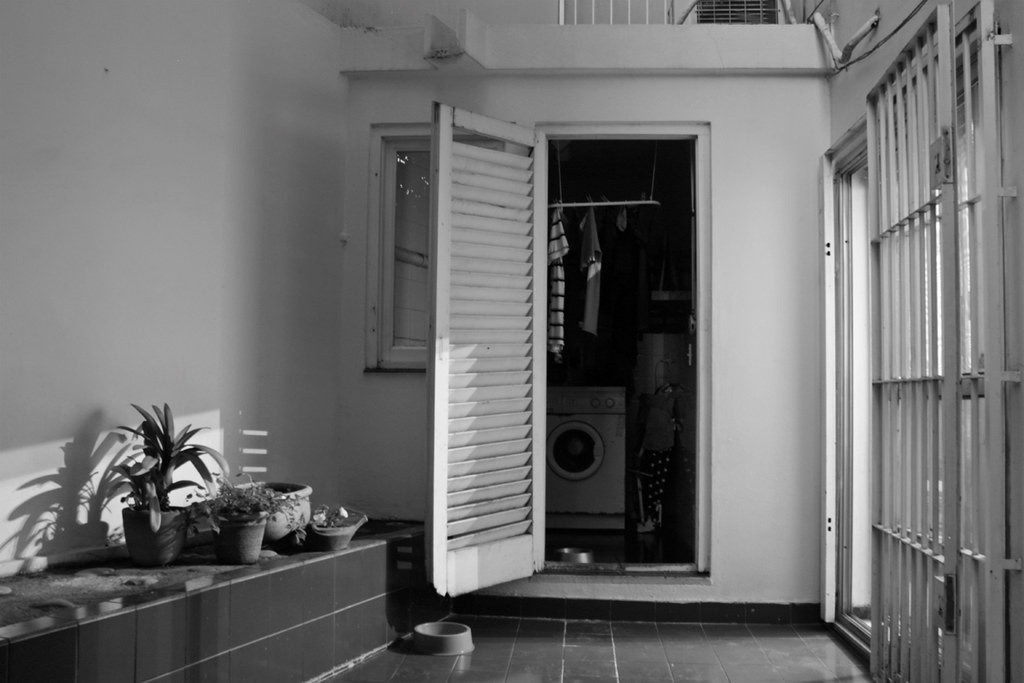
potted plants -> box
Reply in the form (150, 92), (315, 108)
(110, 403), (368, 567)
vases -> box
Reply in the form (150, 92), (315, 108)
(235, 482), (313, 540)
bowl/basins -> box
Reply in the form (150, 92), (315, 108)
(413, 622), (475, 658)
(556, 546), (594, 562)
(233, 481), (314, 545)
